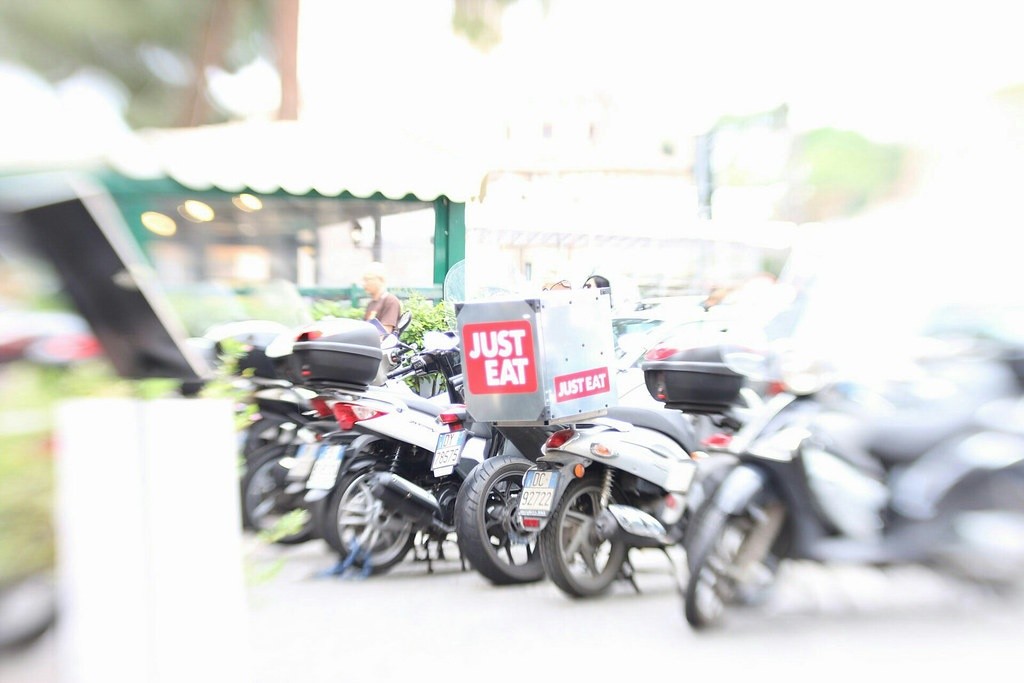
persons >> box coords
[581,274,612,308]
[354,260,402,335]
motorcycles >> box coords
[229,283,1023,636]
